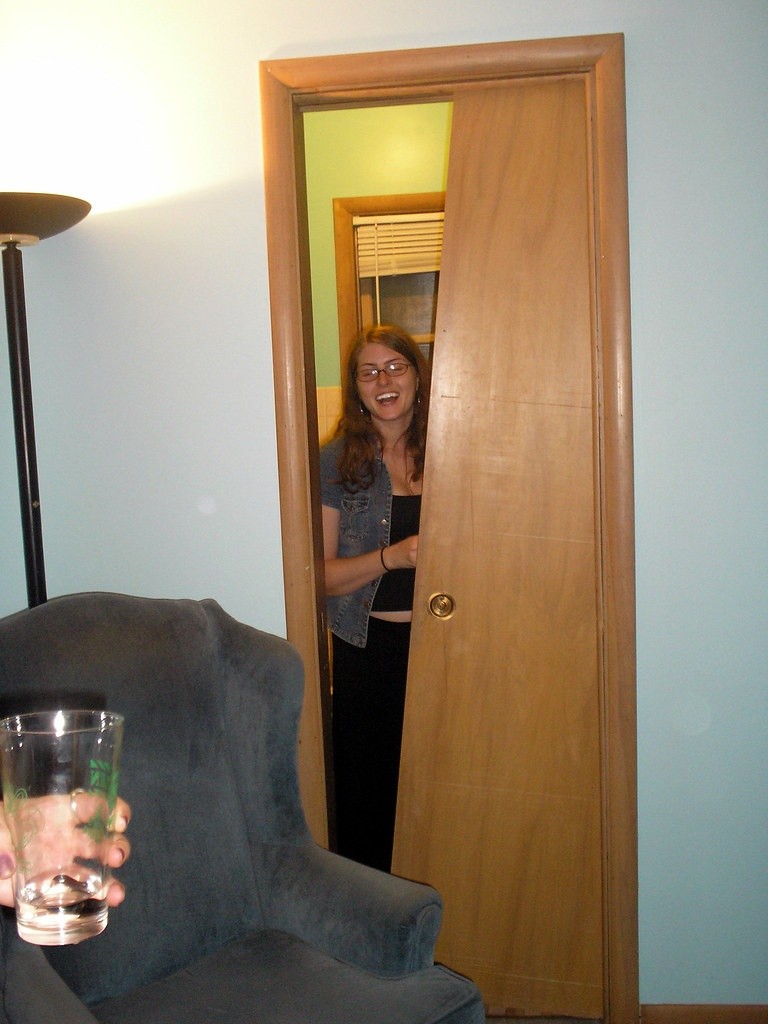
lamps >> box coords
[0,192,90,611]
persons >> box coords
[0,787,133,917]
[316,324,429,875]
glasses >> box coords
[356,362,412,382]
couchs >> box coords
[0,593,485,1024]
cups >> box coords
[0,710,125,945]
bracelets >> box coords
[379,545,392,572]
